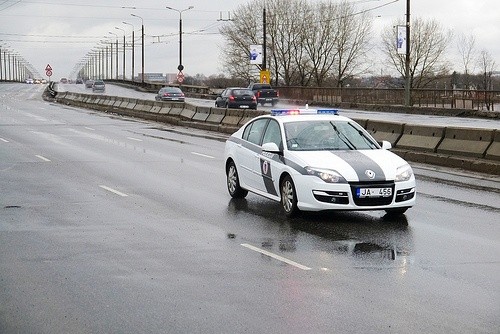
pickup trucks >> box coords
[248,83,280,107]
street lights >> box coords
[123,21,135,81]
[116,26,126,80]
[109,32,118,79]
[74,35,112,80]
[166,5,194,87]
[0,39,40,82]
[131,13,144,82]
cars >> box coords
[85,79,94,88]
[26,78,48,84]
[60,77,83,84]
[92,81,107,93]
[214,86,257,110]
[154,87,185,102]
[223,106,415,216]
